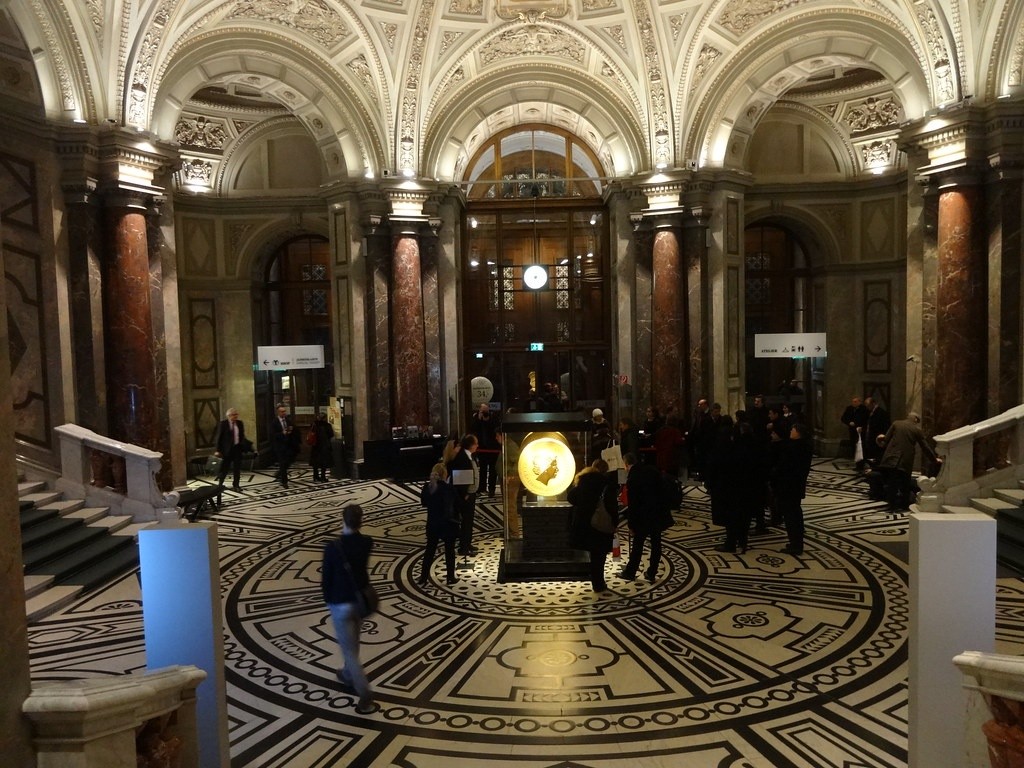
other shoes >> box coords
[356,692,374,709]
[644,571,655,580]
[283,483,289,489]
[713,517,804,553]
[615,572,634,580]
[233,483,242,491]
[459,550,478,556]
[448,578,460,584]
[469,546,478,551]
[274,474,280,479]
[335,670,355,686]
[419,578,427,583]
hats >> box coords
[909,413,921,424]
[592,409,603,418]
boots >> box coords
[313,467,320,481]
[320,469,328,482]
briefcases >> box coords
[206,454,223,474]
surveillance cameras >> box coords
[906,355,914,362]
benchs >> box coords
[174,484,225,520]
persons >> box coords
[419,434,479,584]
[468,405,501,496]
[528,382,559,401]
[215,407,245,492]
[310,413,334,482]
[565,394,812,594]
[274,407,296,486]
[322,504,374,707]
[841,397,938,510]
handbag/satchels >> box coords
[855,432,863,463]
[355,586,380,617]
[591,486,617,535]
[306,422,316,445]
[601,439,625,472]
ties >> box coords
[232,422,235,440]
[282,418,287,432]
